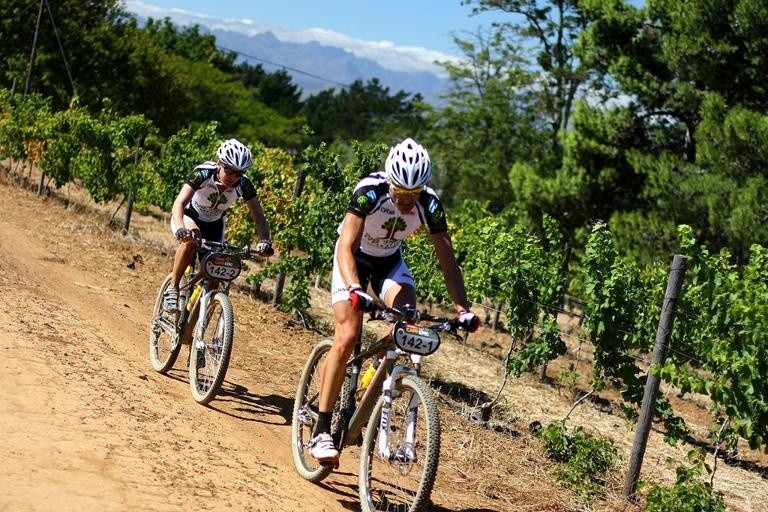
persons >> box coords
[307,137,480,463]
[162,139,274,368]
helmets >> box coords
[215,138,252,170]
[384,138,432,190]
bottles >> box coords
[186,282,202,312]
[359,359,381,401]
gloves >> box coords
[347,284,374,312]
[256,239,274,257]
[175,228,192,243]
[455,310,480,333]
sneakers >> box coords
[197,351,205,367]
[311,433,340,458]
[162,285,179,313]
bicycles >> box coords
[290,285,468,512]
[149,231,272,404]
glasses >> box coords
[221,163,243,177]
[389,179,423,199]
